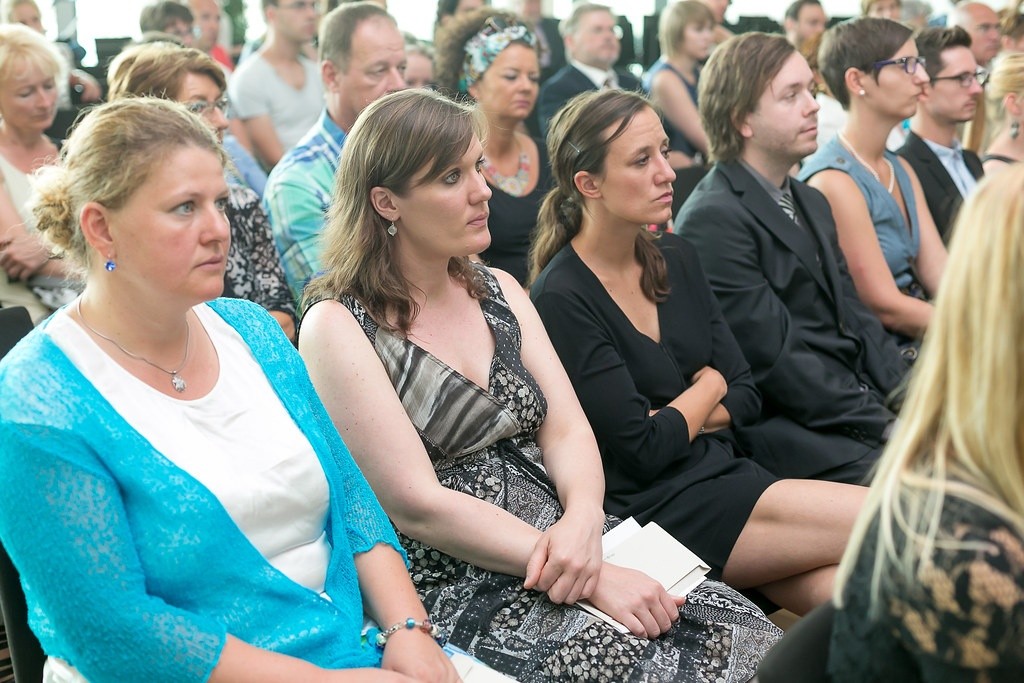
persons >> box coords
[0,0,1024,683]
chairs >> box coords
[0,304,48,683]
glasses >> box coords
[861,57,929,76]
[183,97,229,117]
[936,64,987,88]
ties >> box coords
[777,190,802,226]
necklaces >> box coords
[481,135,530,196]
[836,129,895,194]
[77,296,191,393]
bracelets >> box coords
[698,427,704,434]
[377,618,447,649]
